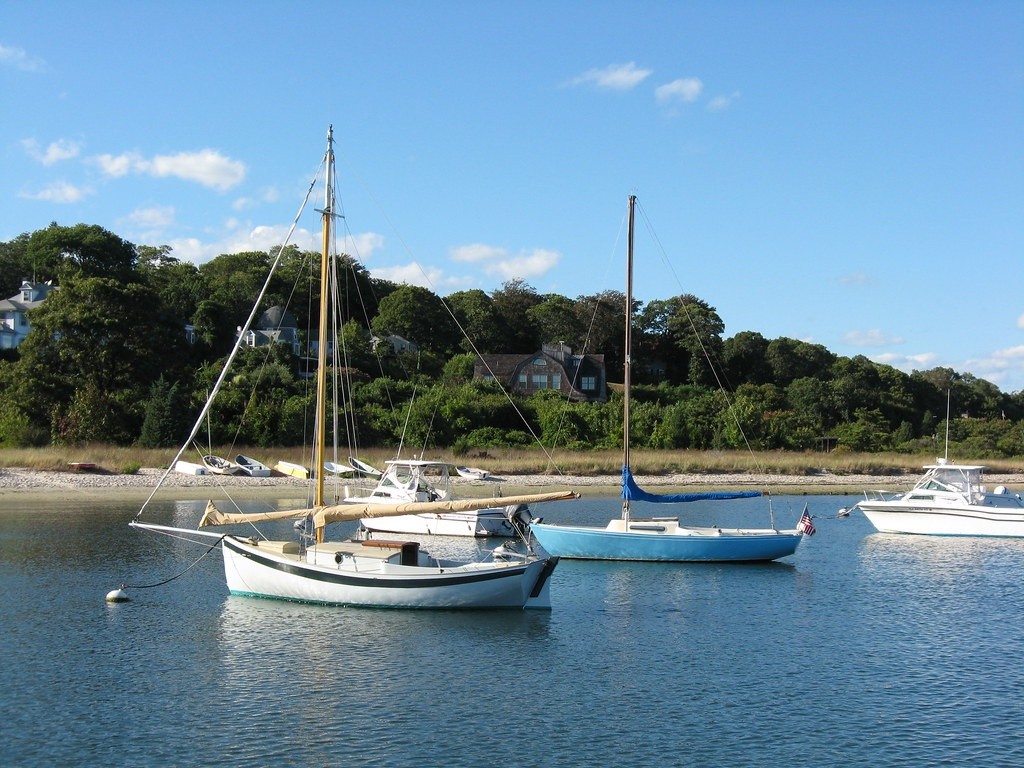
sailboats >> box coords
[526,194,829,562]
[123,123,583,611]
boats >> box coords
[454,466,493,481]
[856,389,1024,540]
[347,456,384,481]
[235,454,271,477]
[323,462,355,479]
[203,455,241,474]
[273,459,310,481]
[175,460,207,475]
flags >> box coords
[798,507,816,536]
